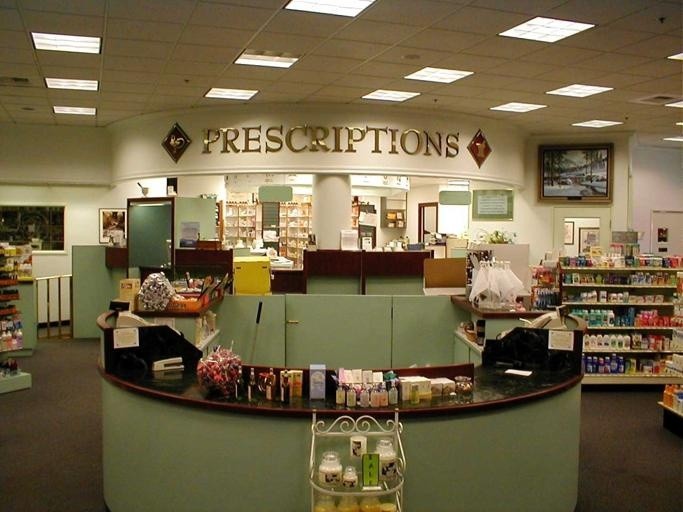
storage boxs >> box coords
[167,276,229,314]
[233,256,272,292]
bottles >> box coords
[572,290,631,373]
[234,365,244,401]
[0,328,25,353]
[342,465,358,490]
[248,367,257,401]
[265,367,276,401]
[335,379,398,408]
[280,370,291,403]
[317,450,344,489]
[663,383,683,414]
[375,438,399,482]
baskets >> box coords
[162,297,205,313]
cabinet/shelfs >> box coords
[558,256,683,387]
[215,200,404,267]
[530,250,558,310]
[424,236,530,296]
[659,273,680,436]
[1,243,34,396]
[308,408,406,510]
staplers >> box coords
[152,357,184,371]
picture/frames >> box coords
[536,141,613,204]
[99,207,127,244]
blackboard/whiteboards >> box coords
[127,204,175,271]
